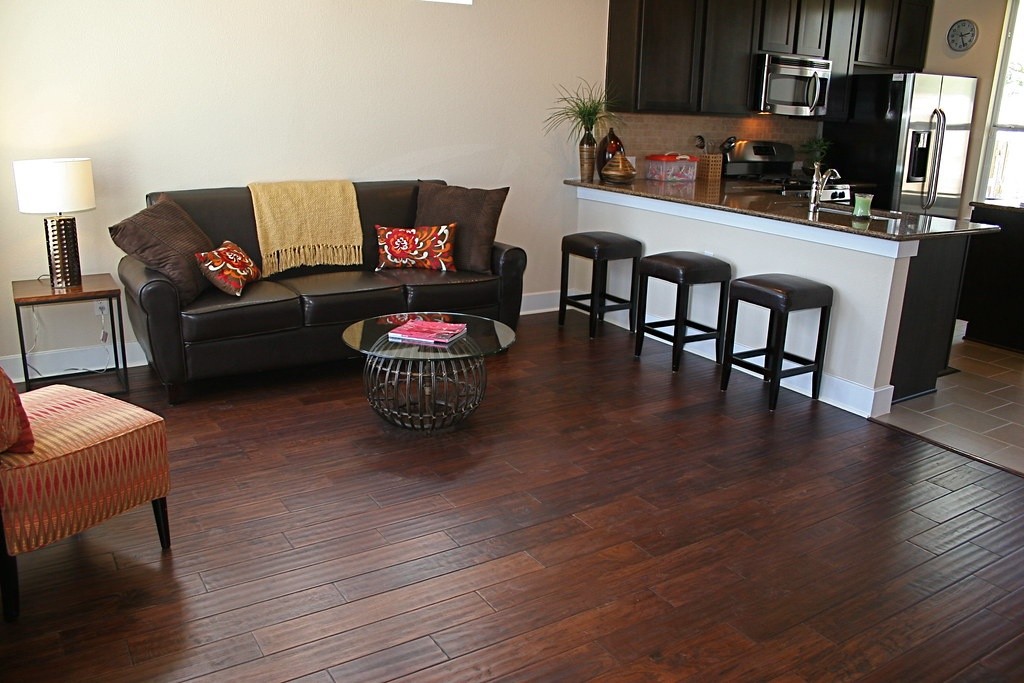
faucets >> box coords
[808,161,841,212]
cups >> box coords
[853,193,874,217]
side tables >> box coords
[14,273,130,399]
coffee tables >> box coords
[342,312,516,432]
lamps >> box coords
[14,157,96,287]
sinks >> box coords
[774,201,913,220]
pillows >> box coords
[197,240,262,295]
[109,192,212,302]
[0,368,36,456]
[414,181,510,276]
[372,223,457,274]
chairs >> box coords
[0,385,172,623]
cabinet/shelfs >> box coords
[858,0,935,72]
[760,0,833,57]
[635,0,756,118]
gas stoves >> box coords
[725,139,851,203]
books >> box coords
[388,319,468,343]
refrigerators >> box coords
[826,72,978,221]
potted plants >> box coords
[543,78,626,182]
[800,136,831,176]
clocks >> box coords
[947,19,978,50]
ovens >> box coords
[752,52,833,117]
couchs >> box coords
[119,180,527,406]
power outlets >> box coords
[94,298,109,316]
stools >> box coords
[559,230,642,340]
[634,251,731,370]
[720,274,834,411]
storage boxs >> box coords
[645,152,699,181]
